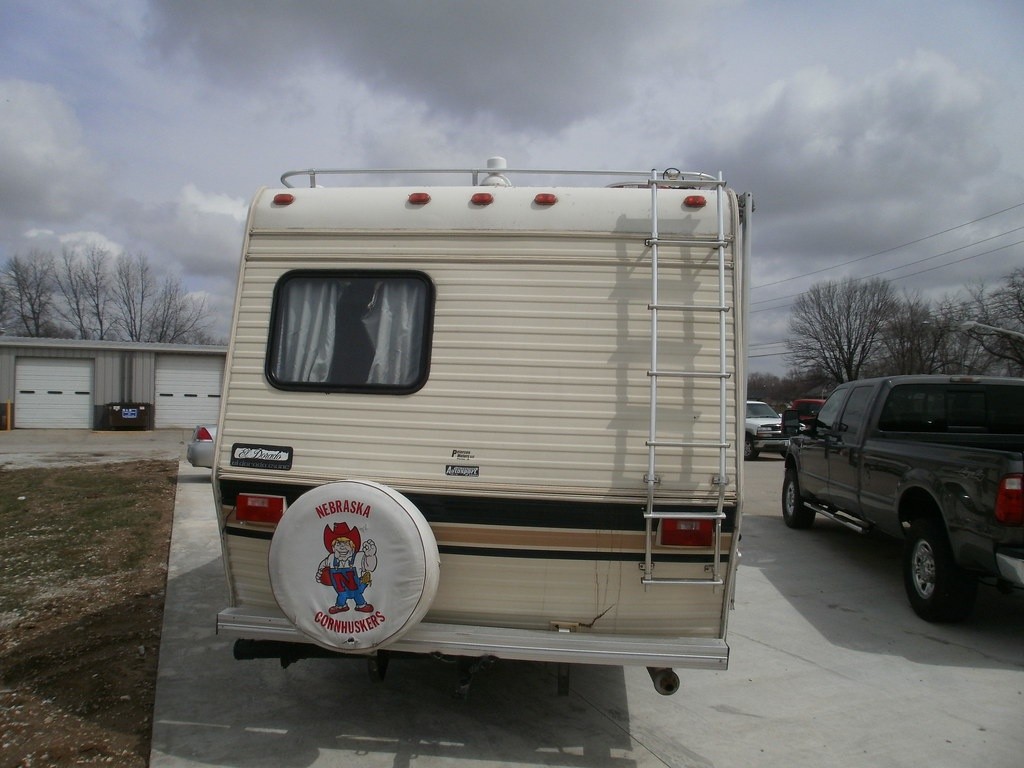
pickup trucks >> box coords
[781,373,1024,627]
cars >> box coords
[745,399,792,461]
[187,423,218,468]
[783,398,829,436]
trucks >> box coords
[212,158,757,701]
